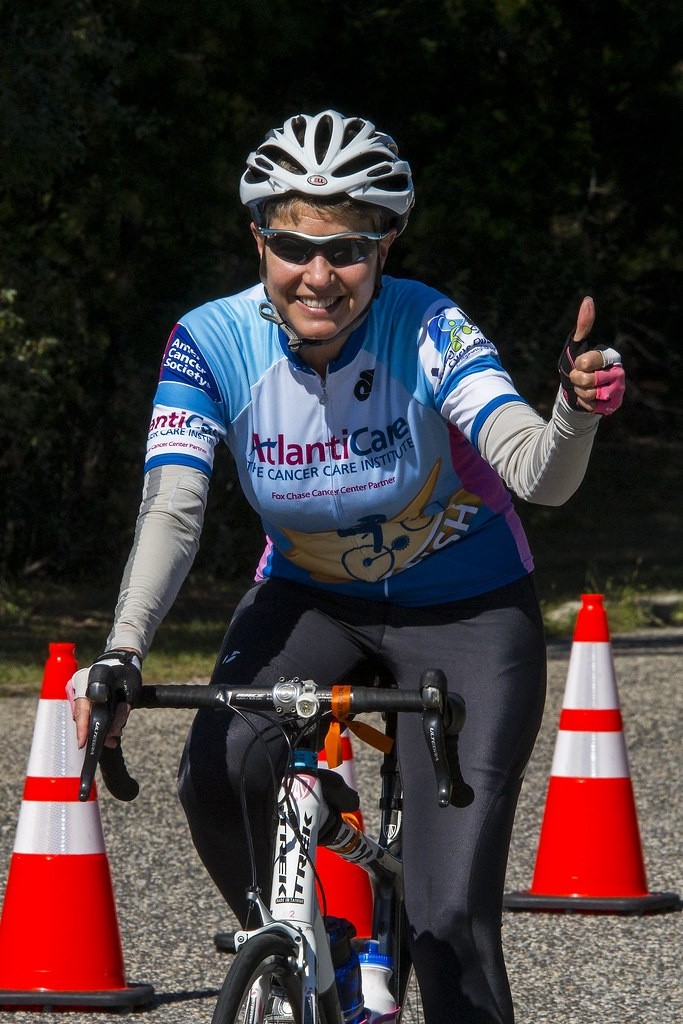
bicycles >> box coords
[78,663,478,1024]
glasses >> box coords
[254,222,390,268]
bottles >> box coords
[358,940,396,1024]
[323,915,364,1024]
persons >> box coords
[64,110,624,1024]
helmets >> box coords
[239,110,416,239]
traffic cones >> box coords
[501,596,681,915]
[0,642,157,1011]
